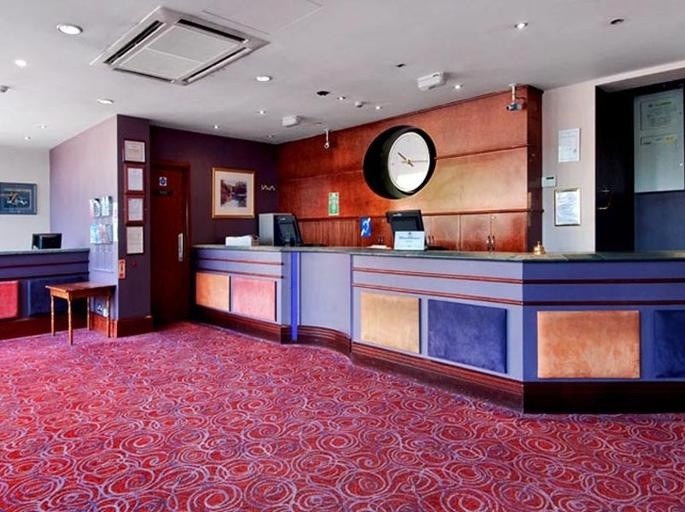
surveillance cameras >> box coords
[505,103,523,112]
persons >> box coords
[222,189,239,208]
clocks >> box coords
[387,130,433,195]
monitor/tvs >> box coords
[386,209,427,248]
[32,233,63,249]
[274,215,305,246]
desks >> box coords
[45,281,116,345]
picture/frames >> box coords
[557,128,581,163]
[553,188,580,227]
[0,183,38,215]
[210,168,255,220]
[120,139,147,256]
